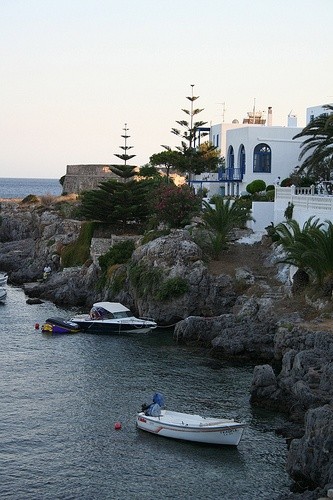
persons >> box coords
[141,393,164,416]
[274,176,280,185]
[43,263,52,282]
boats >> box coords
[70,302,157,335]
[0,272,8,286]
[0,287,7,302]
[136,409,248,447]
